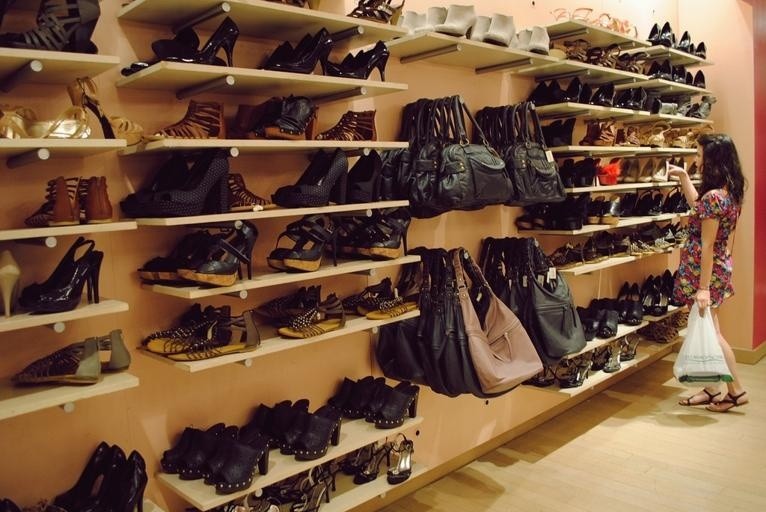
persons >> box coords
[666,133,749,413]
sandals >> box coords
[185,494,283,512]
[0,0,102,55]
[314,109,378,141]
[0,104,92,139]
[277,291,347,338]
[648,189,664,216]
[167,309,262,361]
[346,0,405,26]
[661,187,682,213]
[671,310,690,329]
[10,336,101,385]
[705,391,750,413]
[648,121,671,147]
[655,326,680,343]
[153,99,227,140]
[624,51,650,73]
[23,175,83,228]
[672,131,696,148]
[587,195,606,225]
[600,195,621,225]
[355,215,373,257]
[229,173,277,212]
[253,284,322,319]
[678,388,722,406]
[100,329,131,372]
[565,38,592,63]
[78,175,114,225]
[615,52,631,70]
[597,42,622,69]
[584,45,603,65]
[365,295,419,320]
[140,302,231,355]
[342,276,393,314]
[634,190,652,215]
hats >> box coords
[151,38,228,67]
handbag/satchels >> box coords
[519,236,562,367]
[454,249,520,400]
[415,248,443,395]
[454,246,545,395]
[378,98,446,219]
[375,245,430,387]
[399,95,486,212]
[425,248,461,399]
[442,250,472,395]
[438,94,515,211]
[503,100,568,208]
[525,237,587,358]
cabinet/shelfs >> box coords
[2,0,715,512]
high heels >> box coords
[215,432,274,493]
[66,75,143,146]
[620,332,640,361]
[326,60,344,77]
[434,4,478,40]
[0,249,21,319]
[617,160,632,183]
[637,158,657,183]
[240,399,293,434]
[327,375,375,410]
[668,156,689,182]
[202,428,261,483]
[386,433,415,485]
[176,220,259,286]
[374,385,420,429]
[645,22,662,45]
[652,157,670,182]
[279,461,340,502]
[259,397,310,438]
[626,85,648,110]
[615,281,631,323]
[676,64,688,84]
[393,11,427,40]
[164,16,240,68]
[353,441,395,485]
[160,422,226,475]
[570,157,594,185]
[289,465,334,512]
[534,78,561,106]
[270,148,329,208]
[53,441,111,512]
[626,283,643,325]
[177,424,240,480]
[284,147,349,207]
[534,358,564,387]
[653,283,672,316]
[545,195,576,230]
[370,207,412,259]
[617,87,634,108]
[579,82,593,104]
[127,450,146,471]
[27,236,104,313]
[342,439,379,475]
[558,353,594,388]
[562,77,584,103]
[342,39,391,82]
[625,158,640,183]
[18,239,95,310]
[282,213,340,272]
[267,26,335,76]
[526,79,547,101]
[659,22,674,47]
[562,192,590,230]
[80,443,127,512]
[676,31,692,52]
[362,379,411,421]
[350,148,382,203]
[413,6,448,35]
[150,151,231,217]
[136,229,243,285]
[293,408,344,460]
[641,275,663,314]
[263,94,320,140]
[343,376,385,420]
[267,220,300,272]
[695,41,707,60]
[470,15,492,42]
[527,26,550,55]
[603,337,623,373]
[130,27,201,71]
[694,69,706,88]
[276,403,335,453]
[482,12,516,47]
[658,58,673,80]
[596,157,624,185]
[516,29,532,51]
[597,82,617,107]
[116,459,149,512]
[646,60,661,80]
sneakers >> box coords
[692,95,717,119]
[533,204,550,228]
[630,240,665,256]
[578,123,601,146]
[549,241,584,270]
[599,309,620,338]
[516,214,533,229]
[593,121,617,147]
[651,98,679,114]
